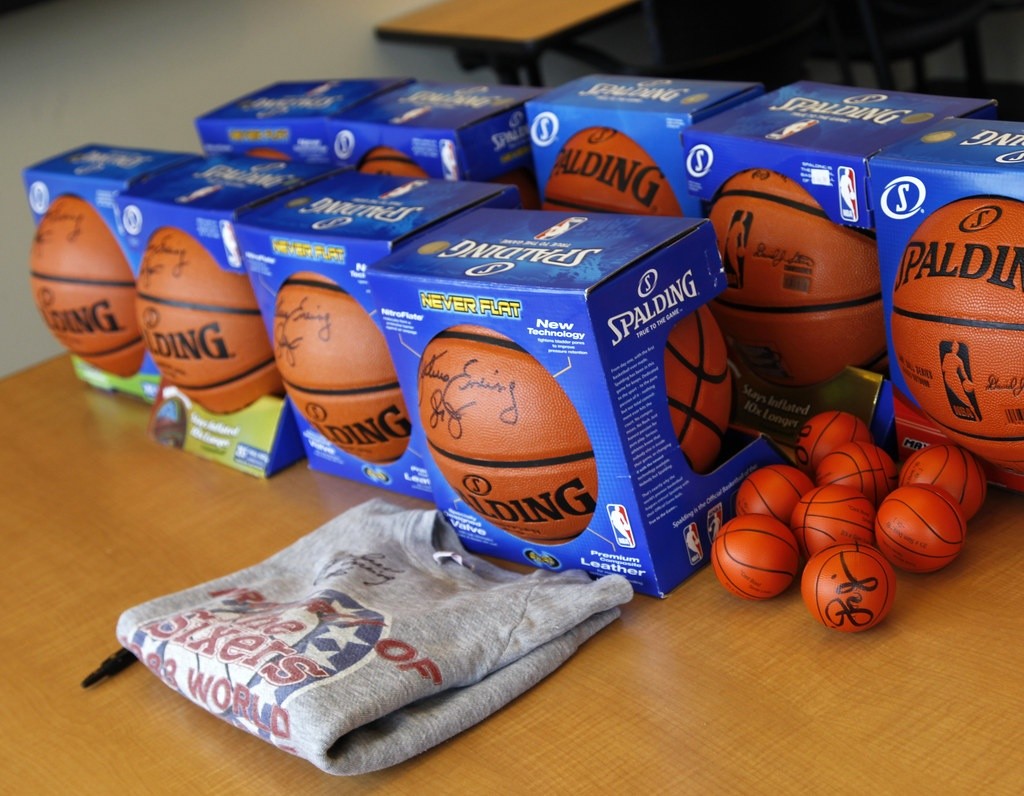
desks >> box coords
[0,350,1024,796]
[375,0,654,84]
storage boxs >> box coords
[230,170,519,502]
[367,210,787,599]
[867,114,1024,494]
[118,155,352,473]
[195,77,417,166]
[678,80,1001,450]
[335,81,541,210]
[24,143,208,402]
[526,73,764,215]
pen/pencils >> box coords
[78,645,138,689]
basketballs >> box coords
[357,146,539,213]
[706,166,891,389]
[890,196,1024,477]
[28,196,149,380]
[710,405,983,635]
[130,229,286,415]
[538,123,689,218]
[375,212,732,548]
[266,267,410,470]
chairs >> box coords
[643,0,820,83]
[810,0,992,95]
